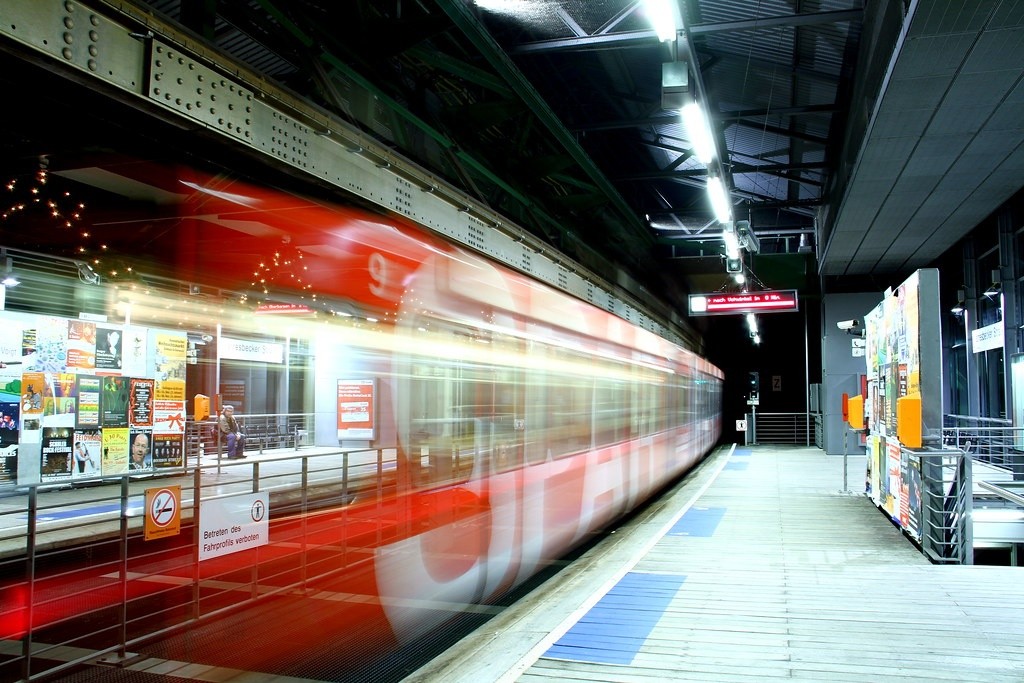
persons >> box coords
[64,400,74,413]
[101,331,121,368]
[219,406,246,459]
[129,433,150,470]
[0,411,17,430]
[75,442,90,473]
[44,400,60,416]
[62,374,76,396]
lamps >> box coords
[660,32,697,109]
[985,286,998,296]
[951,302,964,313]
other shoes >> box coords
[238,455,247,458]
[228,456,238,459]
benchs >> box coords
[210,423,302,460]
